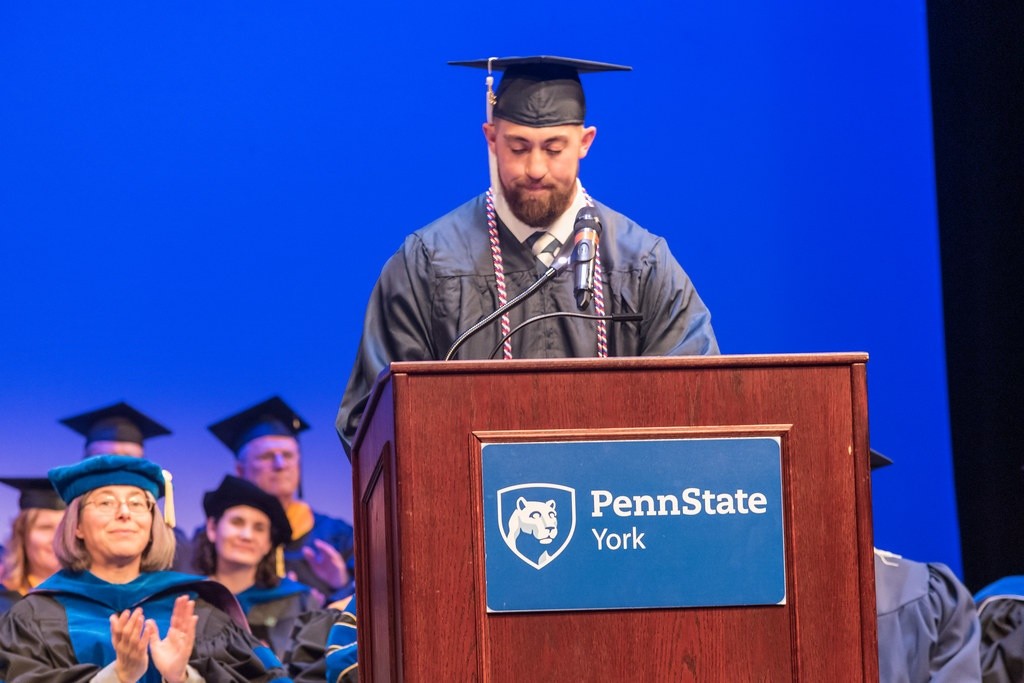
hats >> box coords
[446,54,633,208]
[208,395,310,497]
[59,401,172,447]
[202,474,293,580]
[0,478,69,511]
[47,454,176,526]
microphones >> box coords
[571,206,602,311]
[488,312,645,360]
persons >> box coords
[334,54,720,465]
[0,398,359,683]
[870,452,1024,683]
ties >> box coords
[523,231,562,269]
